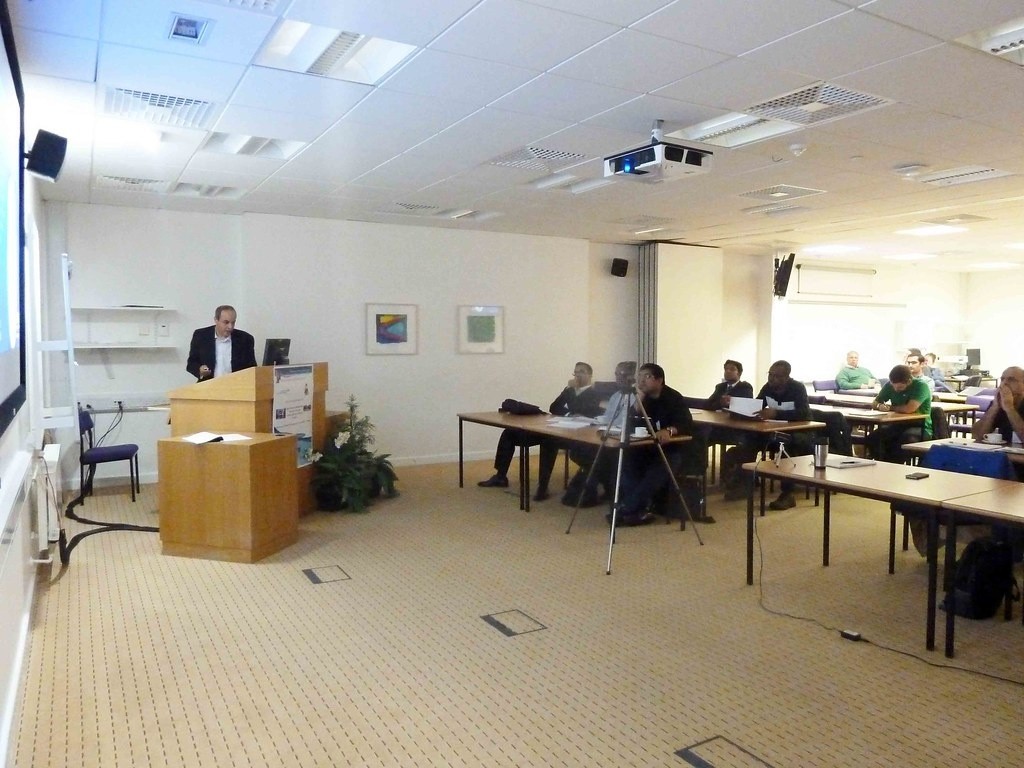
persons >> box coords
[705,359,752,445]
[971,365,1024,445]
[186,305,258,383]
[866,365,933,464]
[905,346,944,402]
[474,362,600,502]
[836,350,881,396]
[563,360,701,528]
[722,360,814,509]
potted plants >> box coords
[317,393,399,512]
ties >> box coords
[728,386,732,389]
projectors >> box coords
[603,141,713,185]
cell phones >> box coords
[905,472,928,479]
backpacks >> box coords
[939,537,1020,619]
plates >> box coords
[631,433,651,438]
[982,440,1007,445]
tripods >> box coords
[565,376,706,575]
[773,441,796,467]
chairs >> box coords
[78,401,140,503]
[588,375,1004,574]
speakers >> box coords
[611,258,629,277]
[26,129,67,182]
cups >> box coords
[983,433,1002,442]
[635,427,648,436]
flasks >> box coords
[814,436,829,469]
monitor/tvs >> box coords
[774,253,795,296]
[262,339,291,366]
[966,349,980,371]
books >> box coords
[723,396,764,420]
[183,432,253,445]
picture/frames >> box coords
[456,305,505,356]
[366,303,417,355]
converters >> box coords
[842,628,861,640]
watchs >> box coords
[876,403,882,409]
[666,427,673,436]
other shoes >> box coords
[477,475,508,487]
[769,493,797,510]
[739,476,759,488]
[606,506,635,526]
[533,488,549,500]
[635,512,655,523]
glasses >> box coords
[615,371,626,376]
[638,374,655,379]
[572,372,583,377]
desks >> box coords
[598,401,827,511]
[458,412,693,531]
[943,375,1000,392]
[806,404,930,469]
[846,390,973,437]
[806,392,979,443]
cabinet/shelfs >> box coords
[894,439,1024,482]
[738,452,1024,668]
[68,306,179,350]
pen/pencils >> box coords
[842,462,858,463]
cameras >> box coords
[616,361,636,376]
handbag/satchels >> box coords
[657,478,703,522]
[561,468,597,507]
[499,399,541,415]
